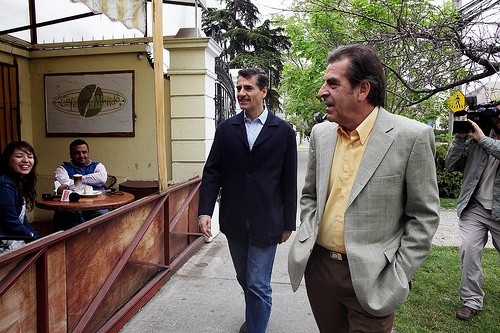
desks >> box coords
[35,190,135,227]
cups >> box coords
[68,193,80,201]
[84,186,93,194]
[73,174,82,186]
[41,193,51,200]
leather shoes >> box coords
[456,305,479,319]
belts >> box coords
[315,244,348,263]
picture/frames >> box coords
[43,69,136,138]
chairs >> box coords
[105,176,117,189]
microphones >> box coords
[453,110,467,117]
[42,189,80,202]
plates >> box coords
[76,191,102,197]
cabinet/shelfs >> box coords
[119,181,159,200]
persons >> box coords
[52,139,109,230]
[197,67,298,333]
[0,140,39,253]
[287,45,440,333]
[445,100,500,321]
